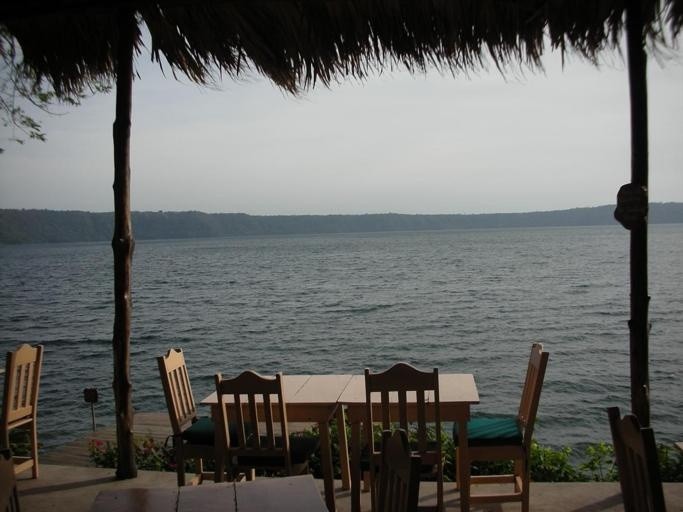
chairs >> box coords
[212,366,312,481]
[361,362,449,510]
[452,342,549,511]
[607,406,666,512]
[0,344,44,512]
[156,346,256,488]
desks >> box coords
[198,374,350,511]
[90,473,328,512]
[337,374,482,511]
